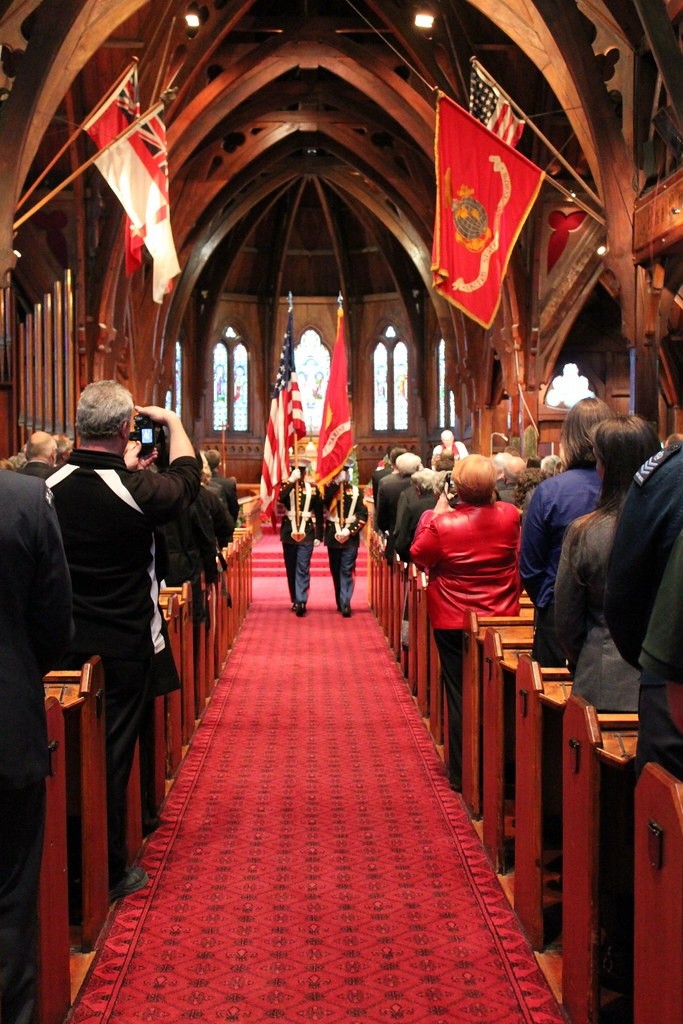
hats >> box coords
[289,458,311,472]
[342,457,354,470]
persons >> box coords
[151,445,240,589]
[601,434,683,780]
[552,416,661,714]
[492,452,562,511]
[324,457,368,617]
[370,443,455,566]
[0,430,69,478]
[277,457,324,616]
[0,471,72,1024]
[431,430,469,471]
[412,454,524,792]
[44,379,202,900]
[519,397,617,669]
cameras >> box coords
[129,414,166,456]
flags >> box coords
[92,103,182,305]
[315,309,355,499]
[431,93,545,329]
[260,311,306,522]
[84,63,145,278]
[469,65,526,149]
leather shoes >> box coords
[292,602,307,616]
[338,606,350,617]
[109,867,151,902]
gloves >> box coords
[289,470,300,483]
[340,529,350,539]
[333,471,345,485]
[313,539,320,547]
